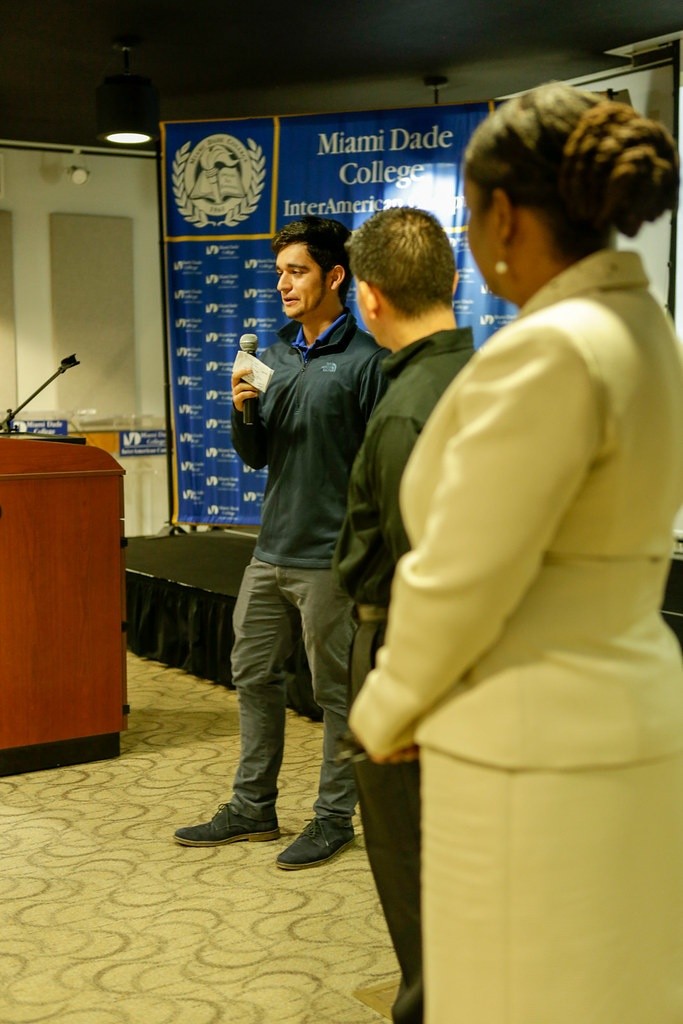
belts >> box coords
[356,602,389,621]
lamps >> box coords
[95,72,158,146]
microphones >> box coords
[239,334,258,426]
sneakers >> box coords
[276,819,354,871]
[173,809,279,847]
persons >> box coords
[175,216,397,872]
[316,204,482,1023]
[350,79,682,1024]
